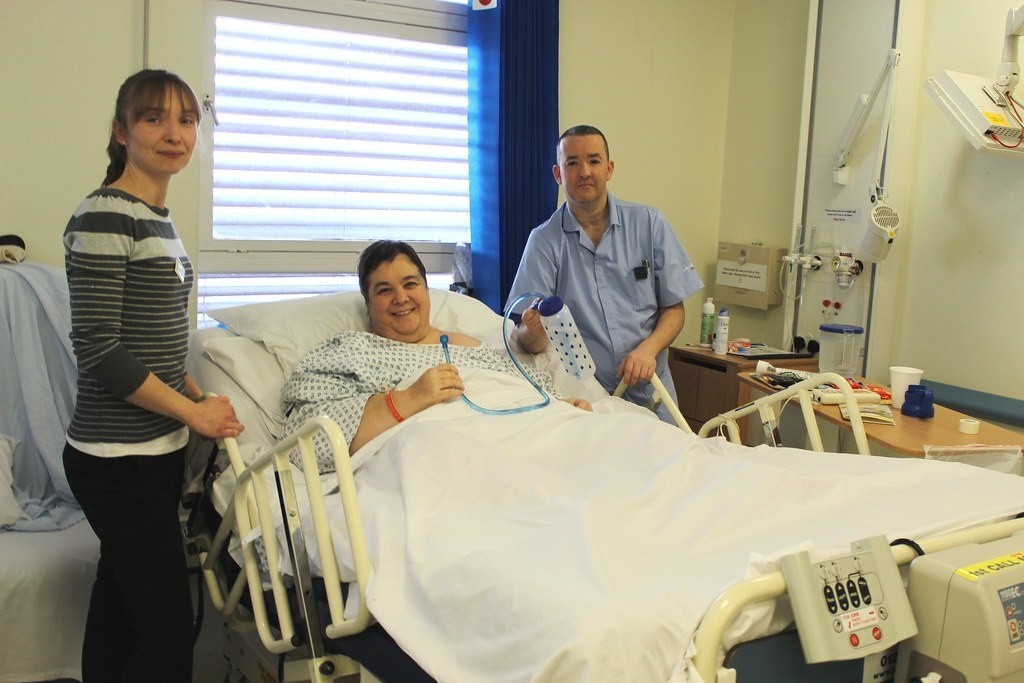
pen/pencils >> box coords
[645,259,651,270]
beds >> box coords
[182,329,1023,682]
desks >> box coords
[736,370,1023,475]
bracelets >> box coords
[385,390,404,423]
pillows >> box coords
[202,330,292,441]
[200,285,514,385]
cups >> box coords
[889,367,923,409]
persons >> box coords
[503,125,706,429]
[279,240,593,475]
[60,68,245,683]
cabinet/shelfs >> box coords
[664,343,820,449]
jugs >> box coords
[819,324,864,379]
